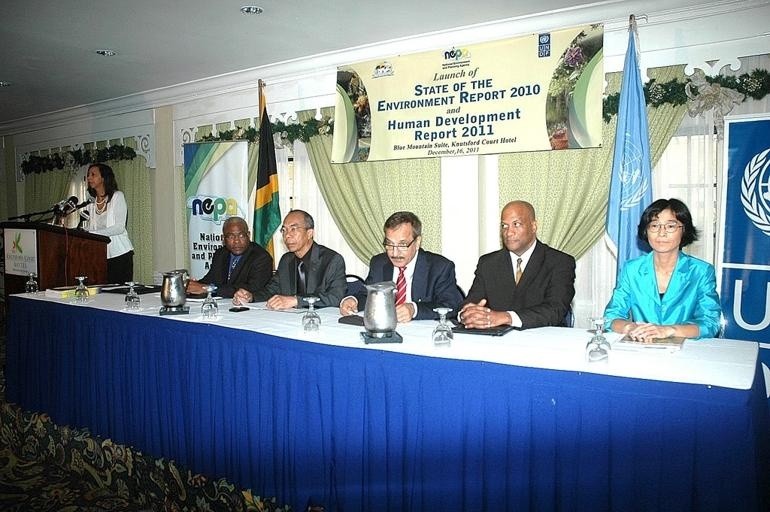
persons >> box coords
[80,163,134,285]
[186,218,273,298]
[340,212,463,322]
[233,210,346,311]
[602,199,722,343]
[454,200,573,330]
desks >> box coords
[6,284,759,512]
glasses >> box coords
[280,227,306,234]
[647,223,682,233]
[222,233,248,241]
[383,237,415,251]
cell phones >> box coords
[229,307,249,312]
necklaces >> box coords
[95,196,106,215]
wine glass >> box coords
[24,271,39,296]
[585,317,613,368]
[200,285,219,320]
[300,296,322,335]
[123,281,140,311]
[431,307,454,351]
[74,275,91,304]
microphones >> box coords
[50,196,92,228]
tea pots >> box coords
[363,279,398,339]
[158,268,191,310]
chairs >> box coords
[345,274,365,296]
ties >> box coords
[230,257,238,272]
[298,259,307,296]
[516,258,522,285]
[395,267,407,306]
